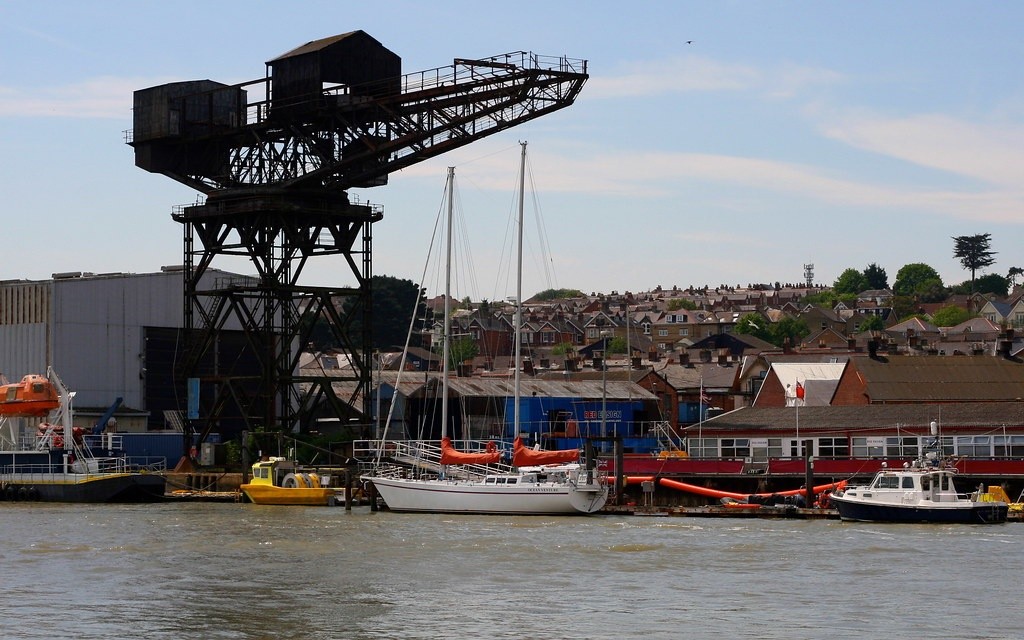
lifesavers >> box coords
[819,493,829,508]
[68,455,73,464]
[190,448,197,460]
[486,441,497,453]
[53,436,63,447]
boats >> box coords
[0,371,61,419]
[827,404,1010,526]
[970,482,1024,523]
[242,435,362,507]
[0,365,168,503]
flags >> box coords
[699,385,712,404]
[797,382,804,402]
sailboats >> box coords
[361,140,610,517]
